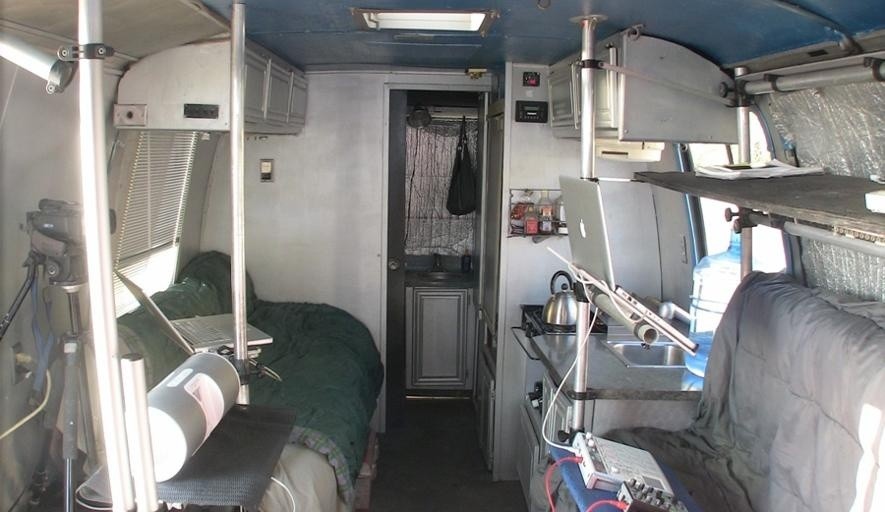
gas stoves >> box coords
[519,304,608,338]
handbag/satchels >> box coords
[447,117,476,215]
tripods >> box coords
[25,332,98,512]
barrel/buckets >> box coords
[682,228,740,381]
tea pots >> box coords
[542,270,579,329]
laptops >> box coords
[558,174,699,353]
[114,268,274,356]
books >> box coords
[695,157,826,180]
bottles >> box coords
[525,389,543,409]
[509,189,570,236]
[461,248,472,274]
